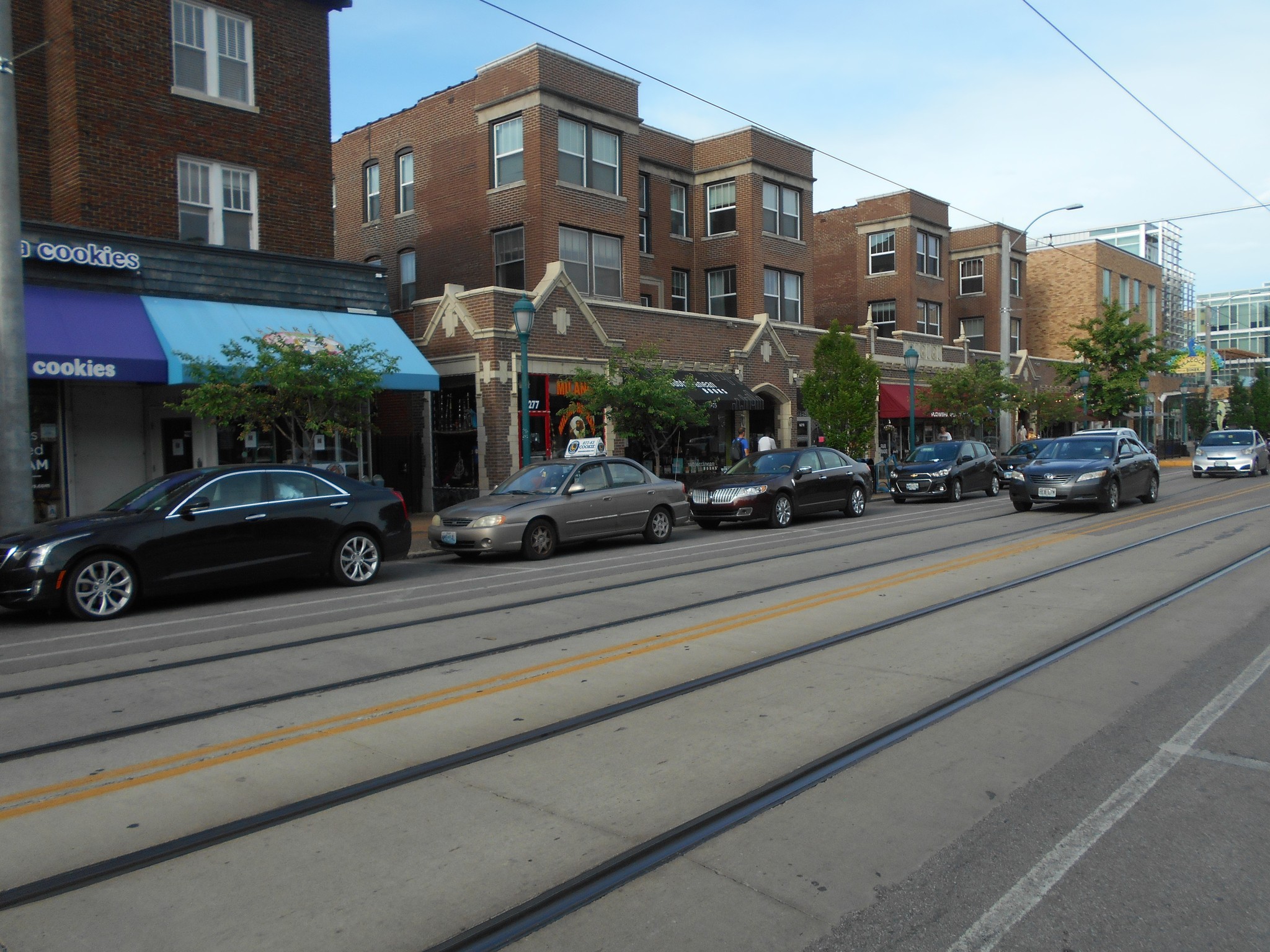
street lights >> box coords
[1000,204,1086,457]
[509,294,544,469]
[903,346,919,456]
[1179,382,1192,445]
[1203,292,1263,435]
[1079,368,1092,429]
[1138,375,1150,443]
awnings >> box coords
[621,366,764,414]
[21,279,441,394]
[878,383,972,417]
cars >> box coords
[1192,430,1270,478]
[998,438,1054,489]
[1010,436,1162,514]
[1,462,413,623]
[426,436,693,561]
[1071,429,1144,460]
[891,440,1000,504]
[687,446,874,529]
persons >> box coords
[938,426,952,441]
[732,427,749,459]
[1017,425,1036,443]
[569,419,586,439]
[758,427,777,452]
